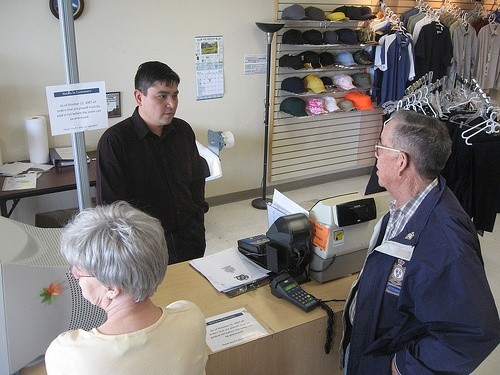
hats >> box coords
[281,4,377,21]
[279,49,374,69]
[280,72,376,92]
[282,28,377,45]
[279,92,374,116]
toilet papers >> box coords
[25,115,49,165]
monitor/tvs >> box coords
[0,216,106,375]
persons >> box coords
[336,110,500,375]
[44,202,208,375]
[94,61,209,267]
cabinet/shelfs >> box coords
[277,18,380,118]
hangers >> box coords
[381,69,500,145]
[379,0,500,44]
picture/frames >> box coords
[49,0,83,21]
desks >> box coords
[0,151,96,218]
[19,261,360,375]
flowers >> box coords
[40,282,64,306]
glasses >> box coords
[75,269,94,279]
[374,137,400,155]
